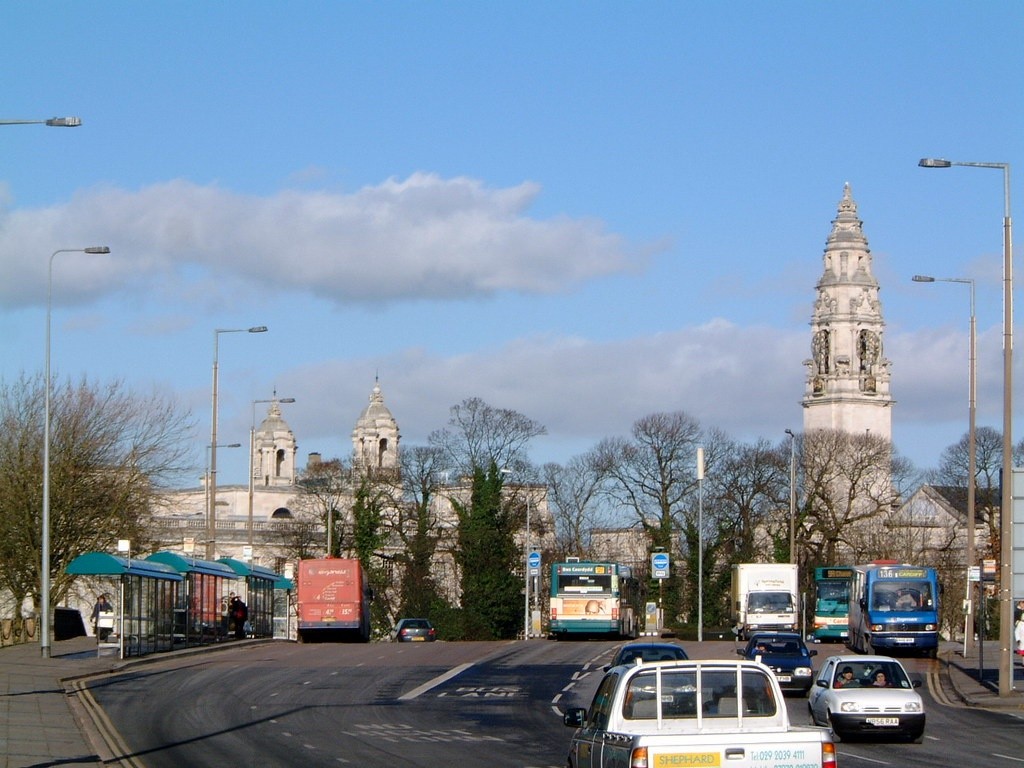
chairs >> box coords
[628,691,658,719]
[717,694,750,718]
[783,642,797,653]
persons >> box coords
[832,666,859,690]
[1014,599,1024,627]
[1013,612,1024,670]
[227,591,236,611]
[756,642,768,653]
[88,595,115,646]
[868,670,896,689]
[229,596,250,640]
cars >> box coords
[605,630,926,742]
[390,618,436,642]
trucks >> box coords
[729,563,804,639]
[294,555,374,642]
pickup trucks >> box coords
[564,655,838,768]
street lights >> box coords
[38,243,296,658]
[782,154,1016,694]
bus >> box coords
[548,557,642,639]
